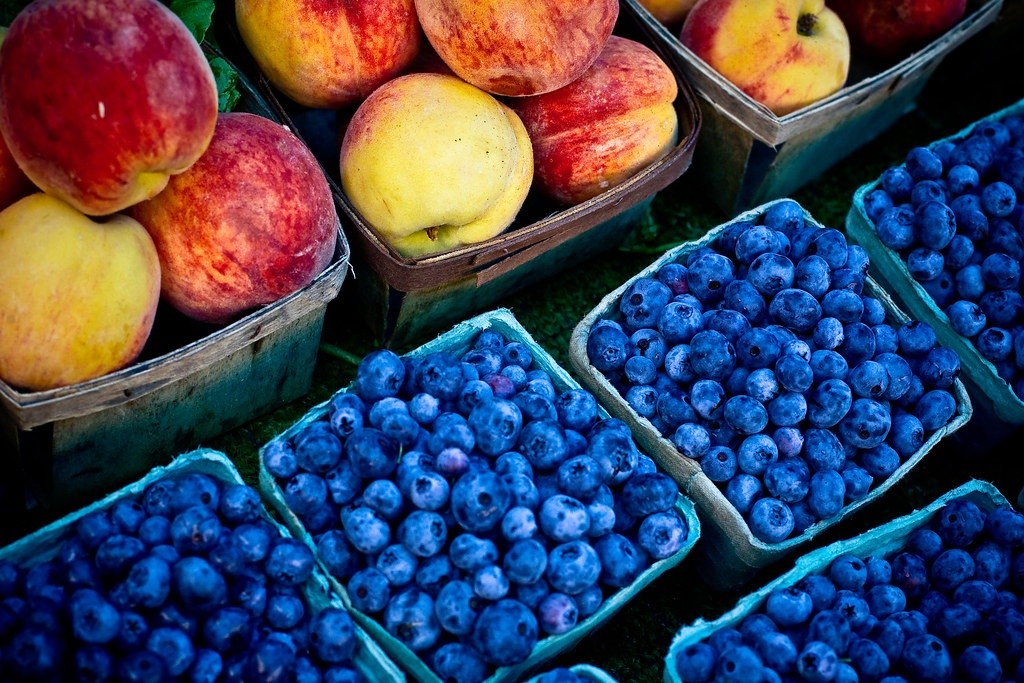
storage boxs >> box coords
[657,477,1024,683]
[524,662,623,683]
[621,0,1006,212]
[222,12,706,356]
[0,38,351,496]
[845,95,1024,425]
[565,190,976,574]
[0,445,409,683]
[256,306,701,683]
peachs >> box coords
[0,0,338,390]
[233,0,678,258]
[638,0,969,117]
